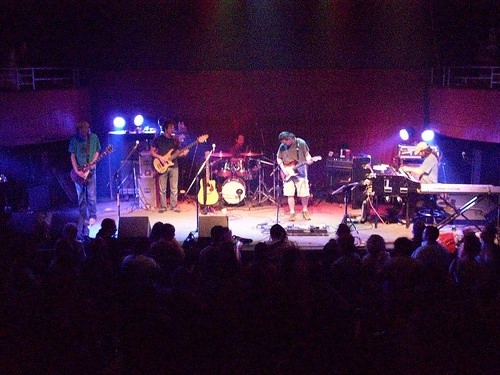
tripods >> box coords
[249,156,277,206]
[332,182,359,234]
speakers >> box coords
[138,152,162,210]
[117,216,151,247]
[199,215,228,244]
[8,210,84,238]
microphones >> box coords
[239,190,241,198]
[212,144,216,152]
[134,140,139,149]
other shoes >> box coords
[302,211,311,220]
[170,206,179,211]
[88,218,96,224]
[289,212,296,221]
[159,208,166,212]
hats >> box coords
[414,141,428,155]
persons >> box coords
[229,134,257,185]
[67,121,101,227]
[277,131,315,221]
[0,209,499,375]
[398,141,440,219]
[150,120,190,213]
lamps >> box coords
[420,126,440,143]
[398,126,416,141]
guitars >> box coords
[152,135,208,174]
[197,151,219,206]
[281,156,322,182]
[70,143,113,185]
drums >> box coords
[211,158,233,178]
[236,157,261,177]
[221,176,246,204]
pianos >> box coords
[416,183,500,236]
[359,162,421,229]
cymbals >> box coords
[210,151,234,157]
[238,152,266,157]
